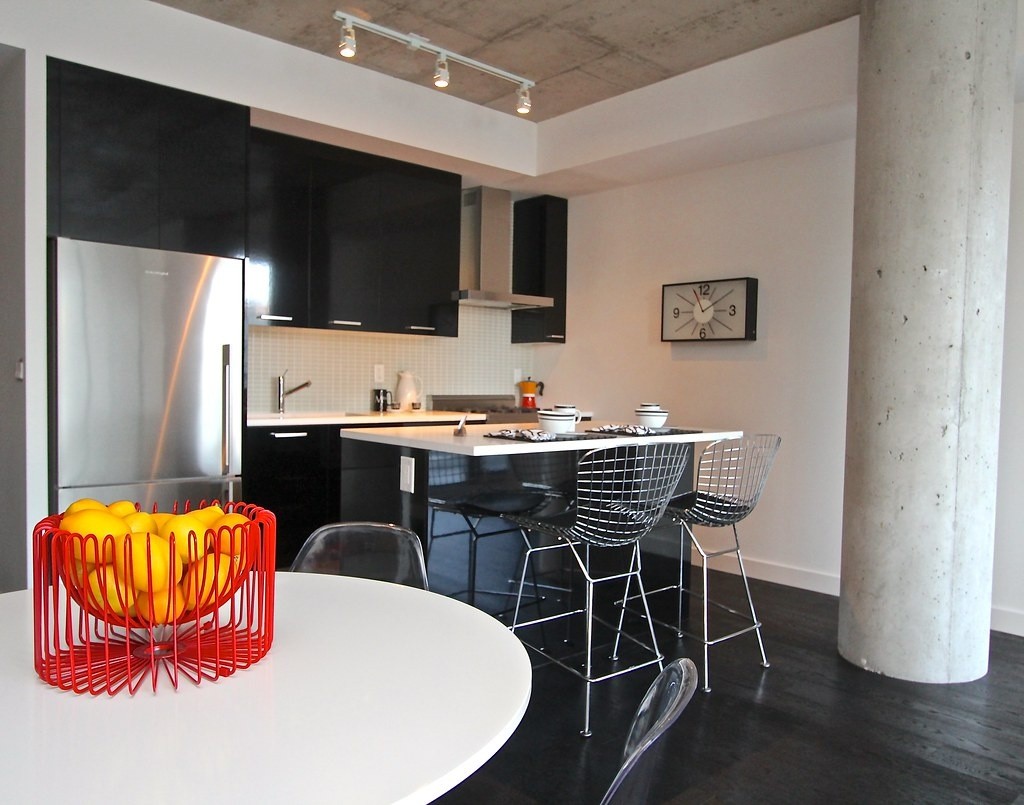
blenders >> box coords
[515,376,544,409]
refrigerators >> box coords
[47,236,245,589]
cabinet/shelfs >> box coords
[48,58,462,345]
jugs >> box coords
[373,389,393,411]
[395,372,423,412]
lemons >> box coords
[57,499,255,623]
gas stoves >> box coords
[427,394,538,424]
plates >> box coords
[649,427,672,432]
[409,410,425,412]
[555,433,589,438]
[386,409,403,413]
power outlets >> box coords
[374,364,385,382]
[514,368,522,384]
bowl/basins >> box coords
[536,410,575,433]
[635,409,669,428]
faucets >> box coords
[278,369,312,414]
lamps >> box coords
[432,53,451,88]
[337,19,358,57]
[515,83,533,114]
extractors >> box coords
[459,186,555,311]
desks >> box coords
[329,422,745,626]
[0,570,536,805]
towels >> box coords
[484,428,556,441]
[584,423,656,436]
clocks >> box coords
[660,275,759,343]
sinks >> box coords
[247,413,373,419]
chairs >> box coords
[290,520,431,592]
[427,472,553,616]
[598,655,700,805]
[508,452,636,593]
[506,440,693,742]
[606,433,783,695]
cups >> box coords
[392,402,400,409]
[412,402,421,410]
[640,403,661,409]
[554,405,582,433]
